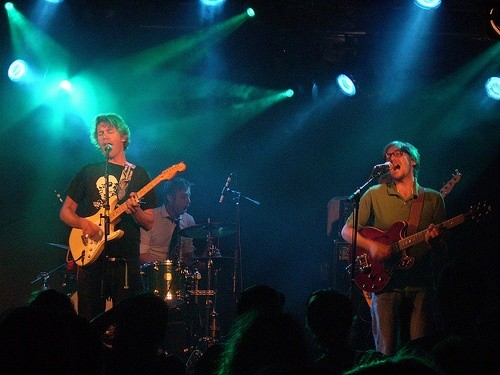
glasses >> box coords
[384,148,409,161]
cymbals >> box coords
[179,220,239,239]
[190,250,234,264]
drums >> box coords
[140,259,187,304]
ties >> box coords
[168,217,181,264]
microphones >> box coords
[104,144,112,151]
[219,173,233,204]
[374,161,392,170]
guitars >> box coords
[343,198,493,294]
[439,168,463,198]
[68,161,187,268]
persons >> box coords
[60,115,159,322]
[0,282,500,375]
[340,141,449,354]
[139,177,205,269]
